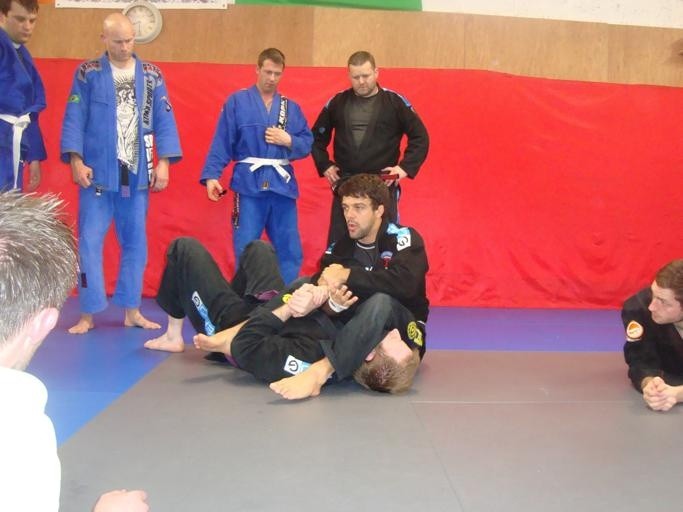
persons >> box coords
[56,11,184,336]
[307,50,432,248]
[0,1,48,196]
[0,184,150,511]
[620,257,683,413]
[190,172,432,400]
[142,237,421,396]
[197,47,315,293]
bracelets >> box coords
[325,297,348,314]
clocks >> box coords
[120,0,163,46]
